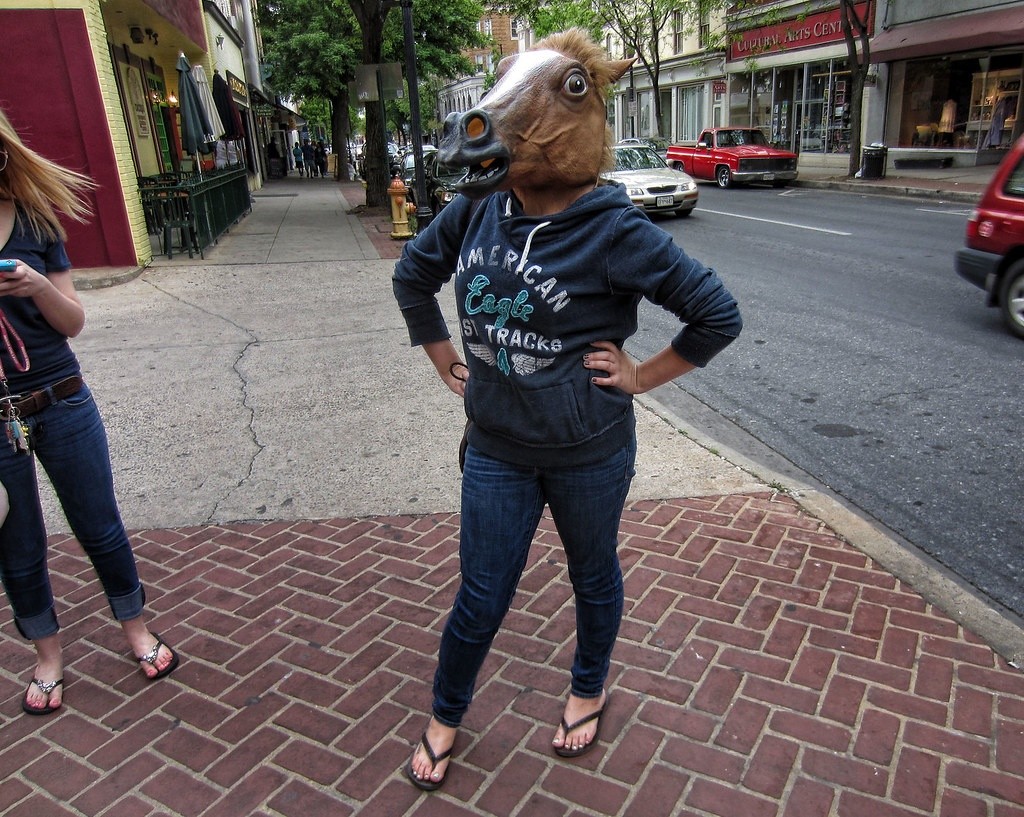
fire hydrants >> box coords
[387,177,417,239]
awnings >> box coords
[857,5,1024,64]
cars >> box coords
[618,138,674,162]
[599,144,699,216]
[349,142,468,218]
[953,133,1024,339]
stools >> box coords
[164,221,199,259]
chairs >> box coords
[136,170,204,235]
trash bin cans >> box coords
[861,145,887,179]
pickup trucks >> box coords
[665,128,798,188]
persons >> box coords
[268,137,281,157]
[938,99,957,144]
[393,29,742,792]
[641,104,649,129]
[0,110,180,716]
[982,97,1008,149]
[213,141,241,170]
[292,139,330,180]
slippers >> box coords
[133,631,180,680]
[406,711,453,789]
[22,664,64,715]
[554,687,609,756]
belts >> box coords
[0,373,83,420]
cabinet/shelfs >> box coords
[821,81,851,153]
[966,69,1024,145]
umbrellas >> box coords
[176,53,225,246]
[212,69,246,169]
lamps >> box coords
[215,32,224,50]
[977,50,990,58]
[645,58,723,83]
[148,87,160,110]
[168,90,178,107]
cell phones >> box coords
[0,260,17,273]
